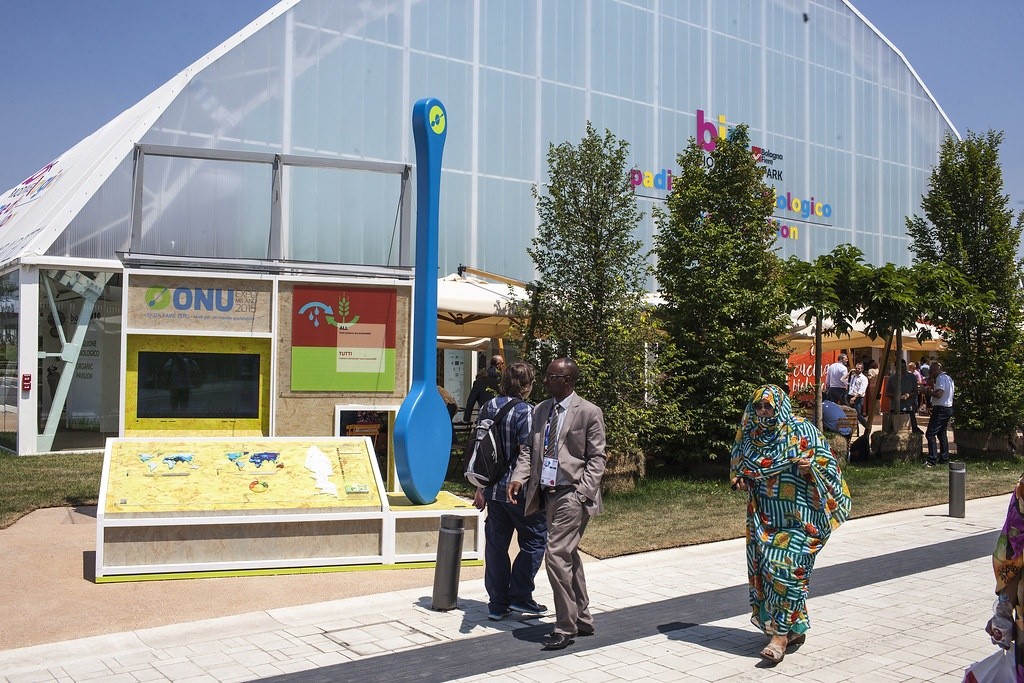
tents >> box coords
[772,310,954,352]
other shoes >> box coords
[924,460,933,468]
[939,459,948,464]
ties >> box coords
[546,403,560,458]
[851,376,856,390]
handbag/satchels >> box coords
[963,643,1017,683]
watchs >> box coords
[999,595,1009,601]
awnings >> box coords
[437,272,593,339]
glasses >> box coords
[542,374,565,381]
[753,402,772,410]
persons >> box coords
[486,354,505,380]
[508,357,607,648]
[730,384,850,662]
[438,386,460,449]
[814,353,956,468]
[463,370,500,422]
[985,471,1024,683]
[472,363,548,619]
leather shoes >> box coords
[577,630,593,636]
[542,633,577,650]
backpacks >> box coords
[464,398,522,489]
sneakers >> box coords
[488,608,511,620]
[509,599,548,614]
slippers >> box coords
[788,632,806,647]
[761,643,785,663]
